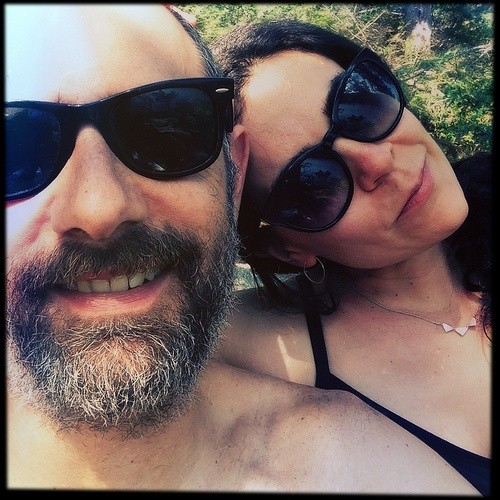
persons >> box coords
[4,4,483,492]
[204,19,495,496]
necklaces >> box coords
[336,266,484,334]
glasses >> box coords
[5,79,235,201]
[247,47,406,235]
